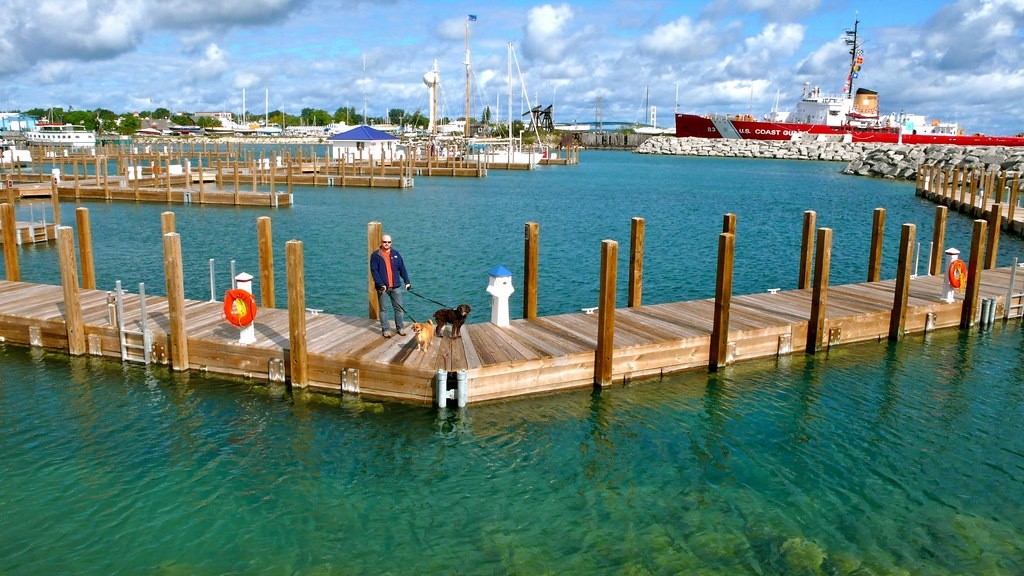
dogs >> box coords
[432,304,472,340]
[410,318,435,354]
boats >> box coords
[26,107,96,147]
[675,12,1024,147]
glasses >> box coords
[382,241,391,243]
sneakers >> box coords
[383,331,391,338]
[397,329,407,336]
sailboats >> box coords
[396,14,545,164]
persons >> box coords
[370,235,411,338]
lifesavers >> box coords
[224,289,258,327]
[949,260,968,288]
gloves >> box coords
[405,283,411,291]
[380,286,386,292]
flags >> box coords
[842,75,852,92]
[467,14,477,21]
[852,56,864,78]
